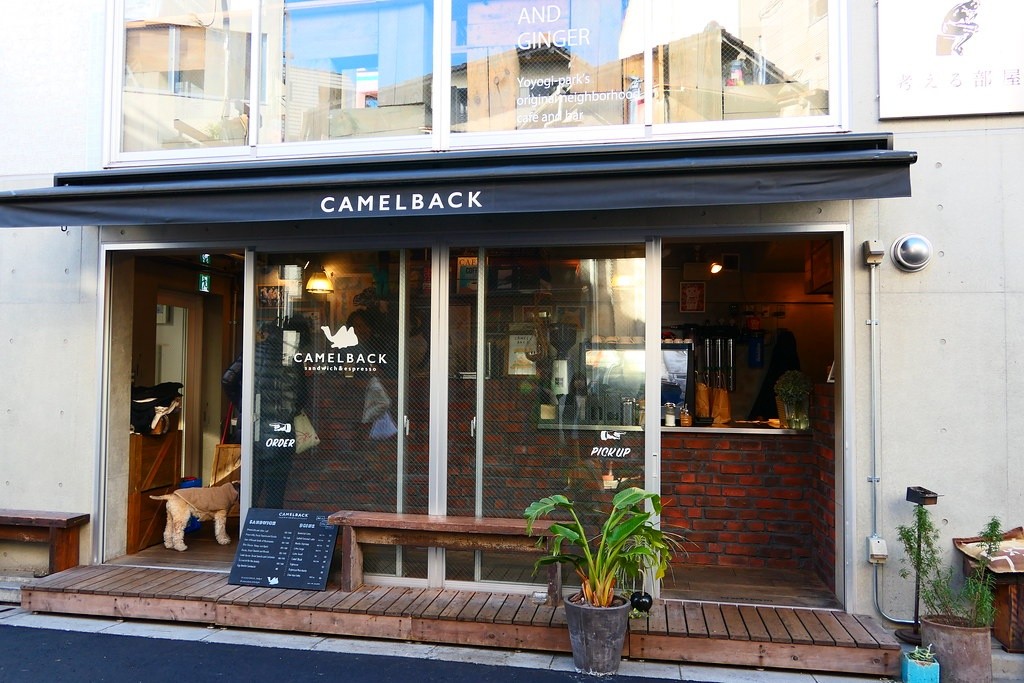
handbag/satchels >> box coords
[294,409,320,454]
[368,410,397,441]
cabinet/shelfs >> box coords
[257,305,325,363]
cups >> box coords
[620,397,635,425]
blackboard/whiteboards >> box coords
[227,506,341,592]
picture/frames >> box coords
[556,305,589,332]
[157,304,170,324]
[521,305,554,322]
[486,304,518,336]
[256,283,288,307]
[679,280,707,314]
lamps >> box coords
[304,264,334,294]
[710,261,723,273]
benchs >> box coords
[0,508,90,575]
[327,512,575,608]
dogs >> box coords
[150,480,242,551]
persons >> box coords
[562,371,603,492]
[219,316,308,509]
[351,365,397,509]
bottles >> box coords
[665,403,676,427]
[639,400,645,425]
[680,403,692,426]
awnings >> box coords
[1,151,918,232]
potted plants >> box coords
[901,643,940,683]
[894,504,1006,683]
[522,487,698,674]
[773,369,815,429]
